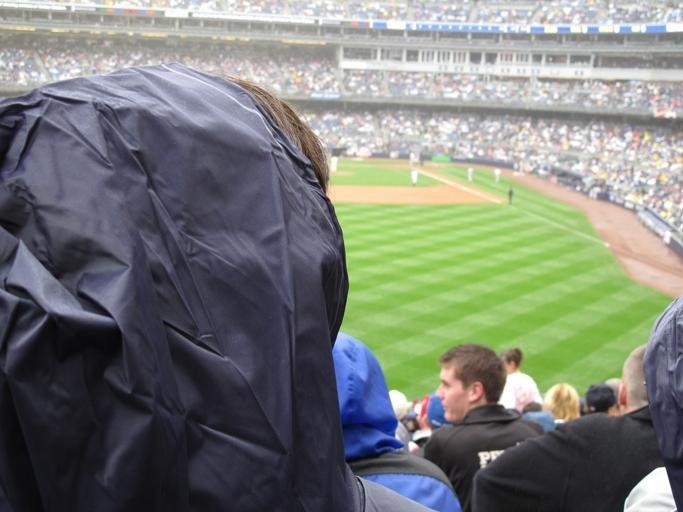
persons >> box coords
[0,0,682,242]
[0,57,429,512]
[621,292,683,511]
[330,343,683,512]
[329,326,461,511]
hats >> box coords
[585,384,614,409]
[389,387,454,429]
